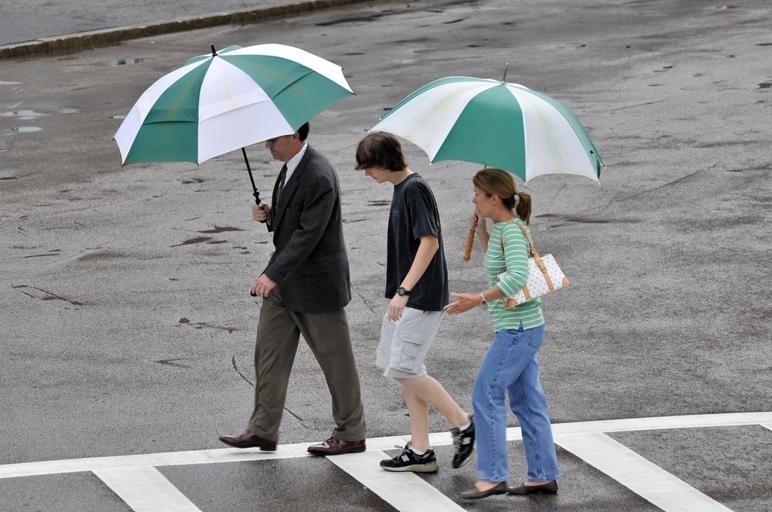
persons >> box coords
[355,131,473,472]
[219,118,366,456]
[442,169,561,499]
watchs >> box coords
[480,292,488,306]
[397,287,411,296]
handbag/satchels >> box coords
[493,217,574,311]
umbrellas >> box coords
[112,42,358,232]
[367,61,609,259]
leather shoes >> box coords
[460,476,510,501]
[306,431,368,457]
[216,425,280,454]
[507,472,562,498]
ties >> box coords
[273,164,291,218]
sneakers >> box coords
[449,412,477,470]
[378,439,442,475]
